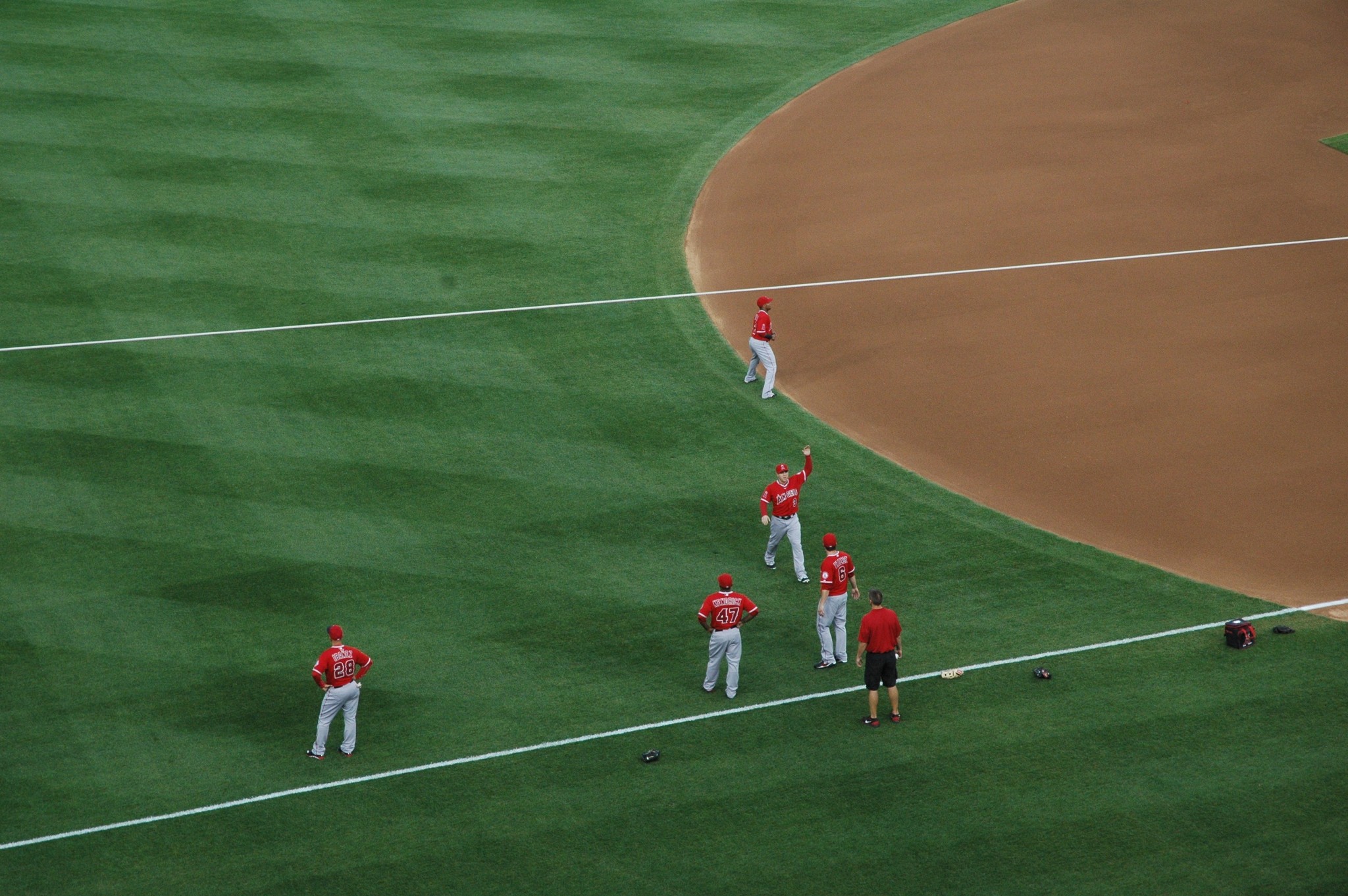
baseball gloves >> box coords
[1033,666,1051,679]
[941,668,964,679]
[641,749,660,763]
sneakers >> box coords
[860,715,880,727]
[305,750,324,760]
[337,746,352,757]
[890,709,900,723]
[834,656,847,663]
[766,561,776,570]
[798,573,810,583]
[814,659,837,668]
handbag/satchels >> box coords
[1224,618,1256,650]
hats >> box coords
[823,533,836,547]
[757,296,774,307]
[327,625,343,640]
[776,463,789,474]
[718,574,732,588]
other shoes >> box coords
[706,689,713,692]
[744,377,758,383]
[762,393,779,399]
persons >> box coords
[698,574,760,699]
[305,625,373,761]
[761,446,813,583]
[856,590,902,728]
[745,296,778,399]
[813,533,860,668]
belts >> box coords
[334,679,353,689]
[775,513,795,520]
[715,626,737,632]
